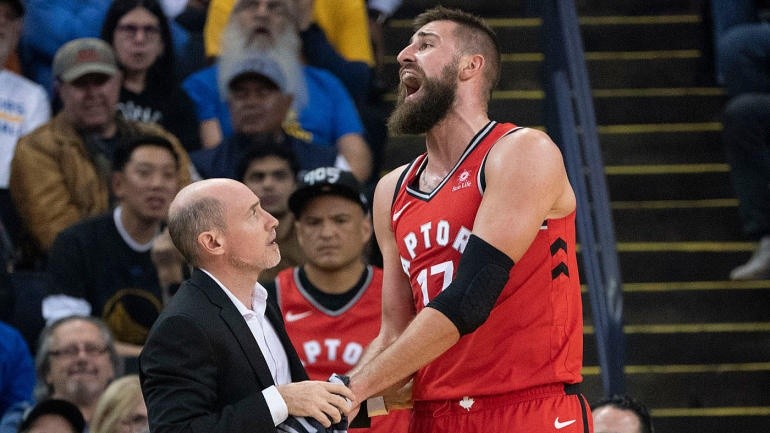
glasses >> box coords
[46,342,113,360]
[115,23,161,41]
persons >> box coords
[273,6,594,433]
[713,0,770,280]
[49,0,197,144]
[589,395,655,433]
[9,37,201,255]
[0,311,148,433]
[0,1,53,273]
[202,0,376,68]
[200,58,358,190]
[192,0,371,182]
[140,176,357,433]
[42,131,199,358]
[263,165,413,433]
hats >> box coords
[52,37,117,82]
[14,400,87,433]
[224,54,287,91]
[287,166,374,211]
